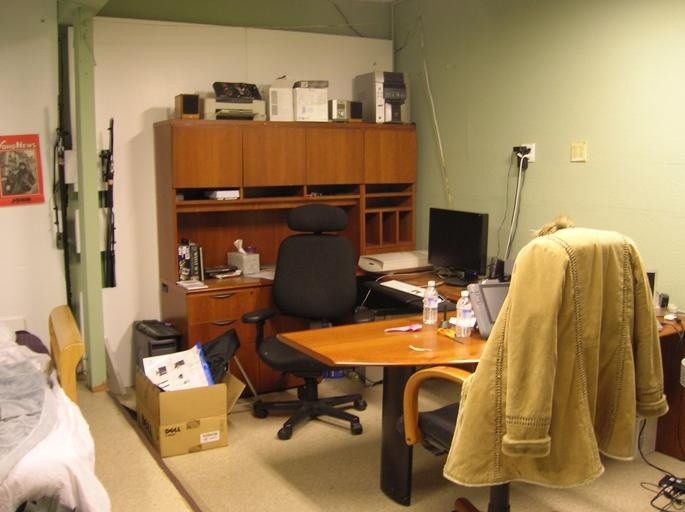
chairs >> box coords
[240,204,375,440]
[404,227,668,512]
[49,304,84,404]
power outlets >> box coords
[518,144,535,163]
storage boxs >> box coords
[135,366,246,458]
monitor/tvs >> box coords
[428,207,489,288]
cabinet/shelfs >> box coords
[153,119,419,400]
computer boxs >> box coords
[135,319,179,371]
[354,303,424,387]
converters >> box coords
[512,145,526,154]
[517,156,527,169]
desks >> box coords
[276,271,685,507]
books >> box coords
[176,238,241,291]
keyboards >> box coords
[137,320,182,340]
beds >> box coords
[0,318,112,512]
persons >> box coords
[6,161,18,194]
[15,163,35,194]
